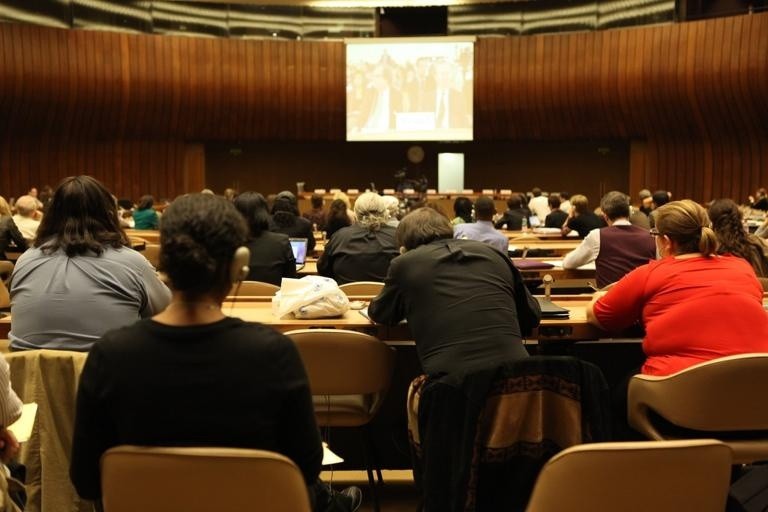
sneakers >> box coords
[341,486,362,512]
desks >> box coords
[511,256,596,293]
[508,240,582,255]
[268,191,525,223]
[221,293,646,342]
[125,227,160,242]
[297,257,318,273]
[503,228,560,239]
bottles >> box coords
[521,215,528,231]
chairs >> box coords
[525,438,734,512]
[98,445,312,511]
[5,346,90,511]
[284,328,395,490]
[230,279,281,296]
[627,352,768,464]
[419,356,605,512]
[338,281,386,295]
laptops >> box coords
[289,237,308,271]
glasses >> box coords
[650,228,660,238]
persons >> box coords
[39,185,52,204]
[7,173,173,353]
[382,195,401,228]
[268,190,315,255]
[316,192,400,287]
[12,194,40,239]
[559,191,571,213]
[748,188,767,211]
[0,351,27,512]
[367,206,543,511]
[320,198,351,240]
[234,191,296,287]
[334,192,357,225]
[223,188,239,203]
[132,194,159,231]
[528,187,551,226]
[638,188,654,214]
[346,52,472,130]
[560,190,660,289]
[561,194,605,239]
[68,190,364,511]
[544,196,569,229]
[0,195,26,280]
[29,186,43,208]
[453,197,477,223]
[586,197,766,443]
[452,196,509,253]
[302,192,328,231]
[495,195,532,230]
[647,190,669,228]
[708,197,767,280]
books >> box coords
[536,298,570,319]
